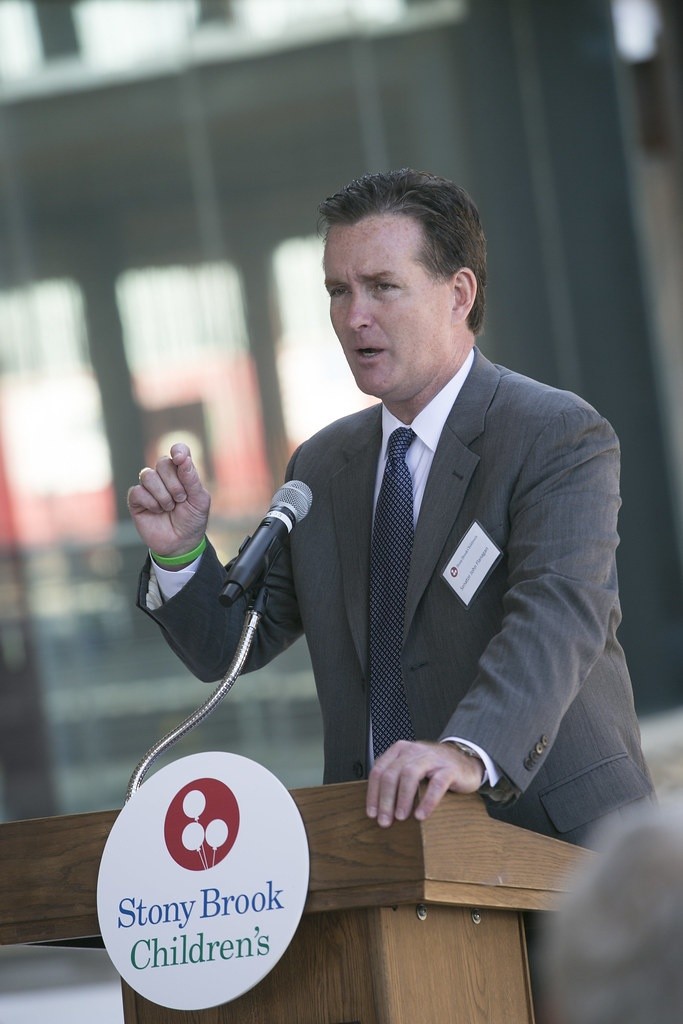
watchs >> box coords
[453,739,482,760]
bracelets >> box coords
[151,535,207,566]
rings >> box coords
[138,467,151,487]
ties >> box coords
[368,427,416,761]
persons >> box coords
[127,168,665,856]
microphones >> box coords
[217,480,313,607]
